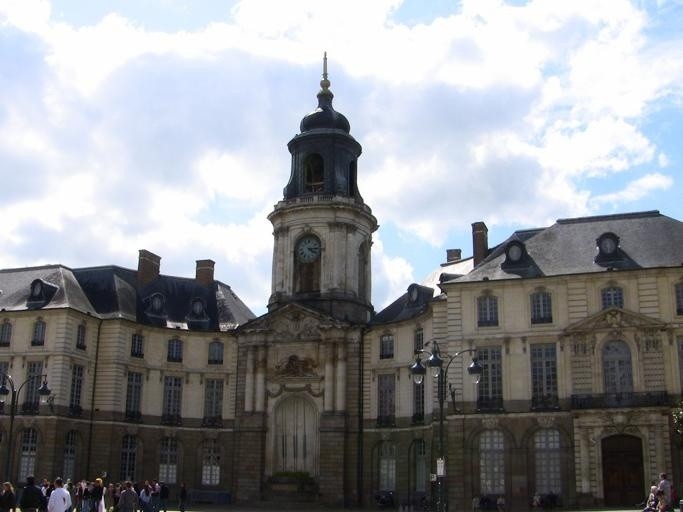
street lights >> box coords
[411,340,482,512]
[1,371,52,482]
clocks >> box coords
[294,235,320,265]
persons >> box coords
[640,472,678,512]
[532,491,543,509]
[178,481,189,512]
[496,494,506,512]
[471,494,489,512]
[547,491,558,506]
[0,475,170,512]
